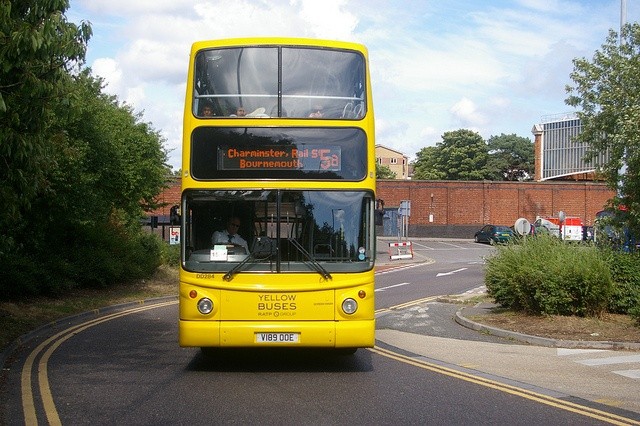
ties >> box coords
[227,235,235,242]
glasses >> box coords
[314,110,323,113]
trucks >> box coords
[531,217,584,245]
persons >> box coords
[306,104,326,118]
[211,218,250,255]
[229,105,249,117]
[199,101,216,117]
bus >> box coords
[170,36,387,359]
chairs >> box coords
[314,244,332,261]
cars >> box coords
[584,226,593,243]
[474,223,514,246]
